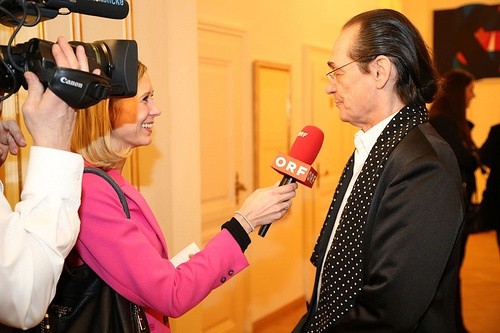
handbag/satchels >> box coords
[41,167,151,332]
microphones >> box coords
[257,126,324,237]
[47,0,129,19]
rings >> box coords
[284,202,290,210]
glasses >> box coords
[325,55,374,85]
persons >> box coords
[66,61,298,333]
[429,69,481,196]
[0,35,101,331]
[477,122,500,246]
[292,9,472,333]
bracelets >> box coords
[234,211,254,232]
